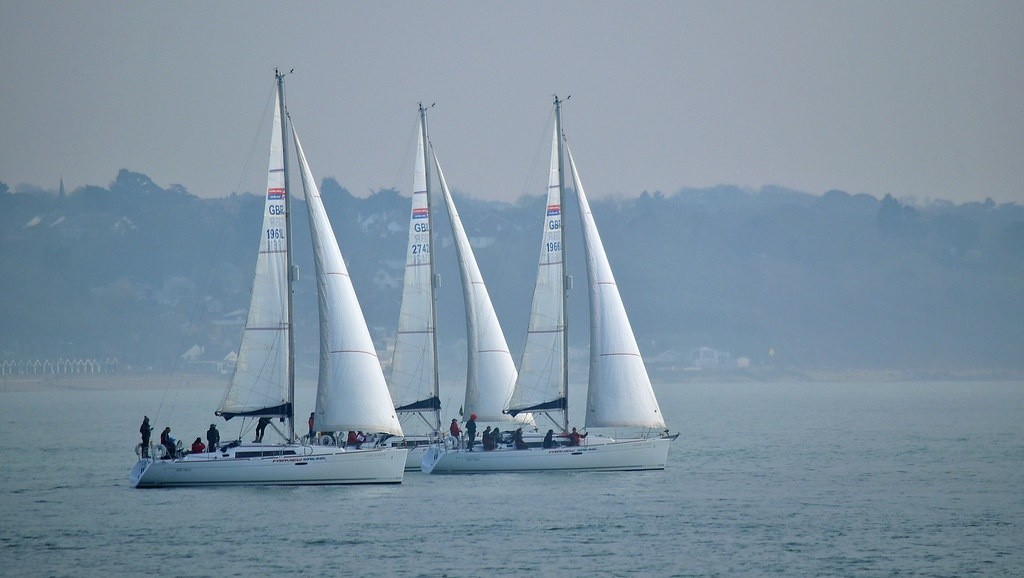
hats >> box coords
[144,416,149,422]
[452,419,457,422]
[470,414,478,420]
[166,427,171,431]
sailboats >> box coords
[383,98,542,473]
[420,94,681,475]
[130,66,412,488]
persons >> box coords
[348,430,367,450]
[191,438,206,453]
[514,428,529,450]
[482,426,502,451]
[139,416,153,458]
[252,416,272,443]
[207,423,220,453]
[308,412,315,433]
[465,414,478,452]
[560,427,588,448]
[450,419,463,449]
[161,426,176,459]
[543,430,557,448]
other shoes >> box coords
[466,447,469,449]
[252,439,262,443]
[469,450,473,452]
